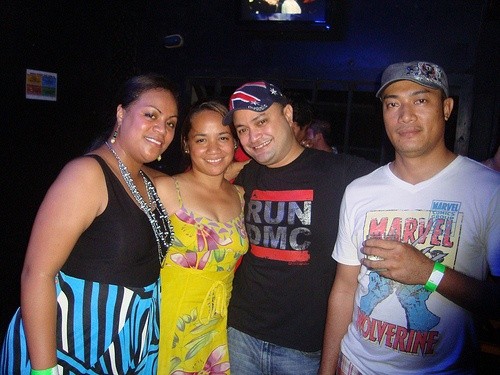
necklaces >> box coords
[103,138,176,269]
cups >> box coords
[366,234,399,272]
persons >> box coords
[305,118,339,154]
[152,100,250,375]
[233,101,316,162]
[315,62,500,375]
[0,73,180,375]
[220,79,357,375]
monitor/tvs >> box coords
[231,0,337,33]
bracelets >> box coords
[422,260,446,293]
[30,364,64,375]
